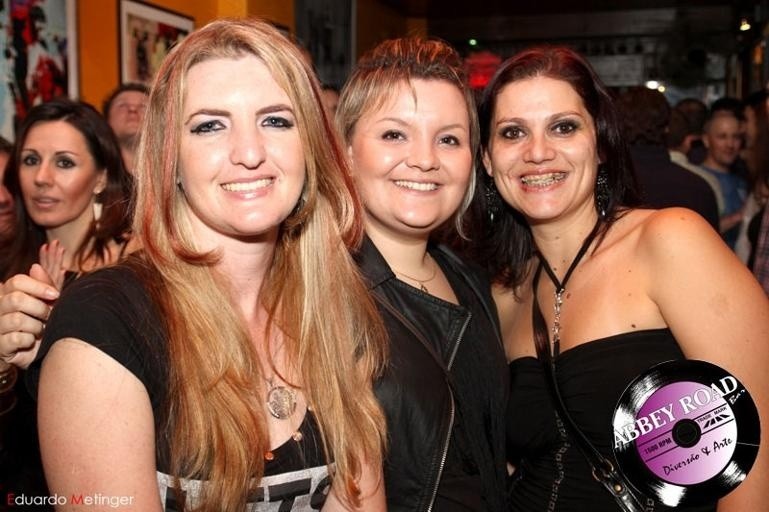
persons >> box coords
[0,11,769,512]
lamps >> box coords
[737,11,752,31]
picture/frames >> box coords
[0,0,80,154]
[118,0,195,93]
